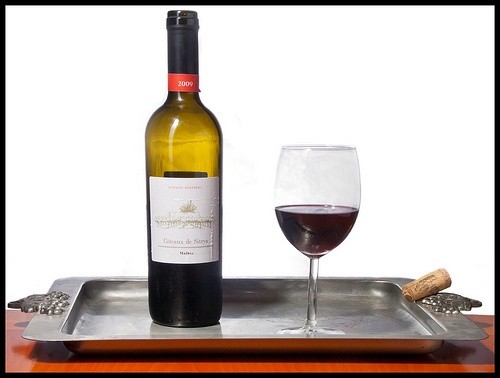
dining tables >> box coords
[4,308,495,375]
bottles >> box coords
[144,10,222,328]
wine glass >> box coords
[275,145,360,336]
